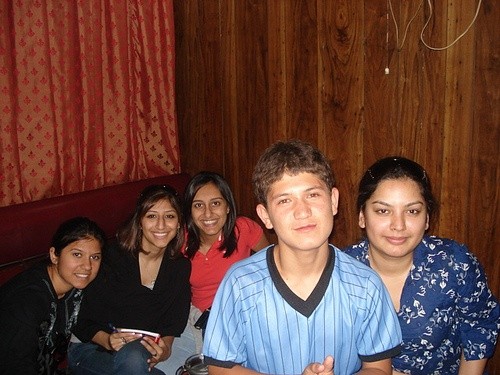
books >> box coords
[113,326,161,345]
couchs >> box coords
[0,173,194,369]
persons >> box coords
[201,140,404,375]
[181,170,270,313]
[65,183,194,375]
[0,214,107,375]
[337,156,500,375]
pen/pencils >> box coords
[110,325,117,333]
[154,336,160,343]
